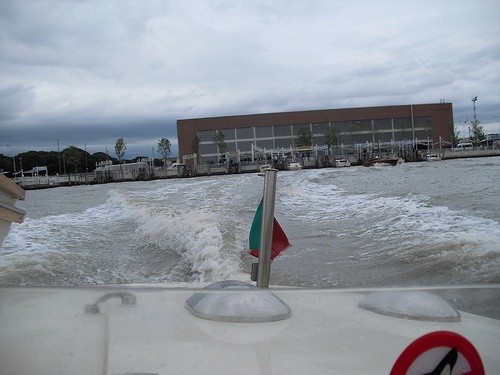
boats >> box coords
[257,136,444,174]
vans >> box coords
[454,142,473,151]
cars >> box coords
[168,162,184,171]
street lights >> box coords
[6,144,16,180]
[472,96,477,122]
